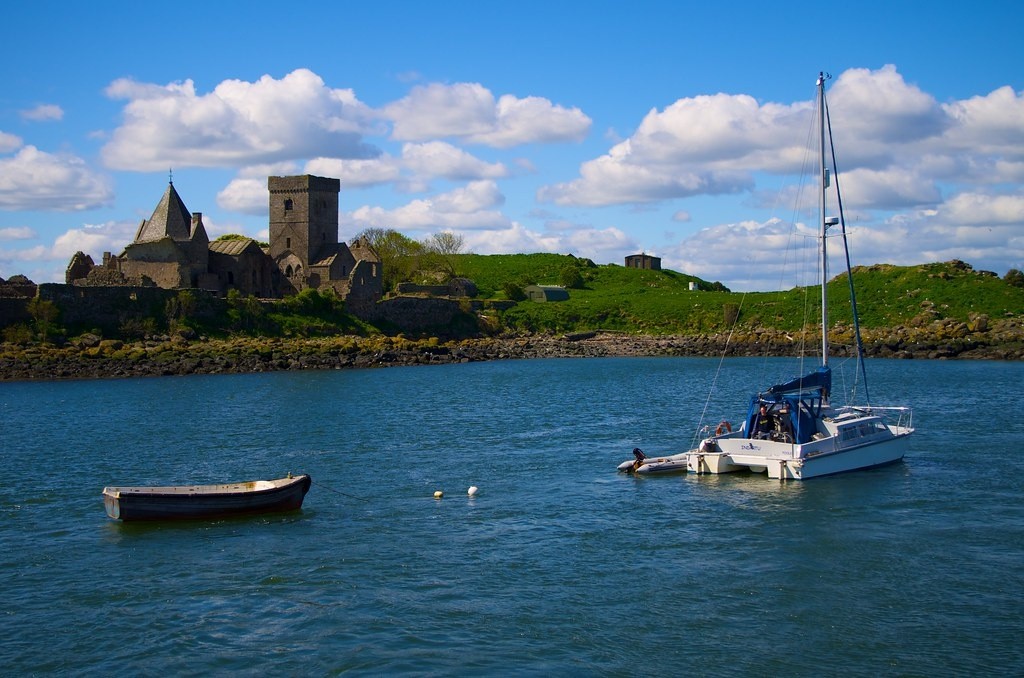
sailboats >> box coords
[686,71,915,481]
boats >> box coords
[616,451,686,476]
[103,473,313,519]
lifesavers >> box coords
[715,420,732,437]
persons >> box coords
[751,405,774,440]
